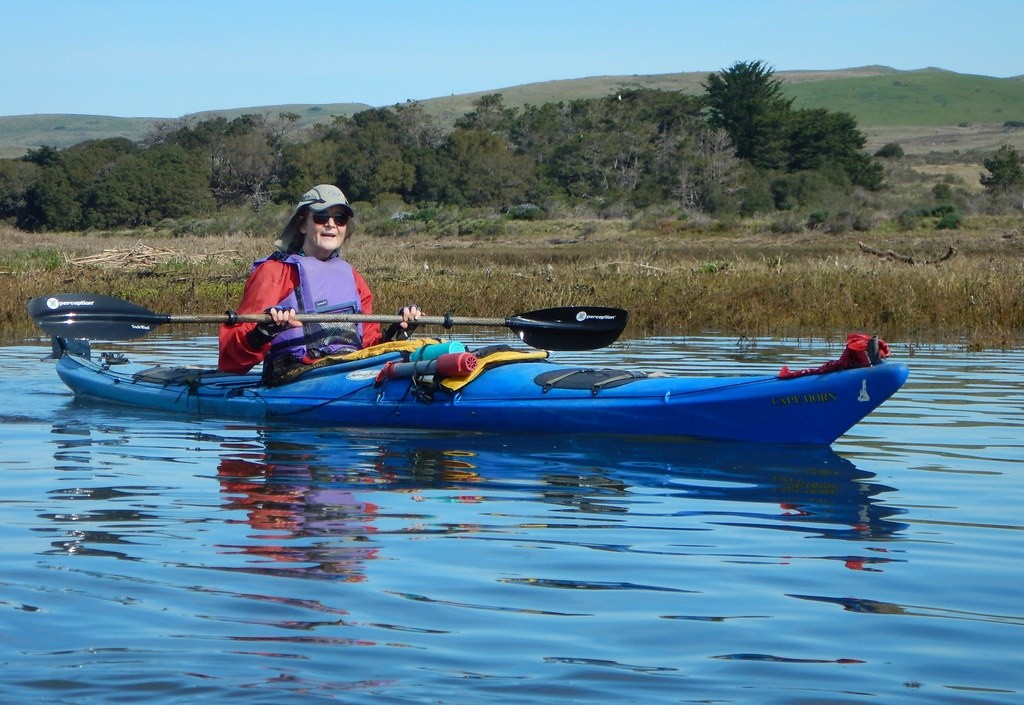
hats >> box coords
[304,184,354,218]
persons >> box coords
[217,183,428,375]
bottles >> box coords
[409,340,466,362]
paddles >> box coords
[28,294,628,352]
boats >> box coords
[41,334,910,464]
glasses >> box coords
[313,210,348,226]
